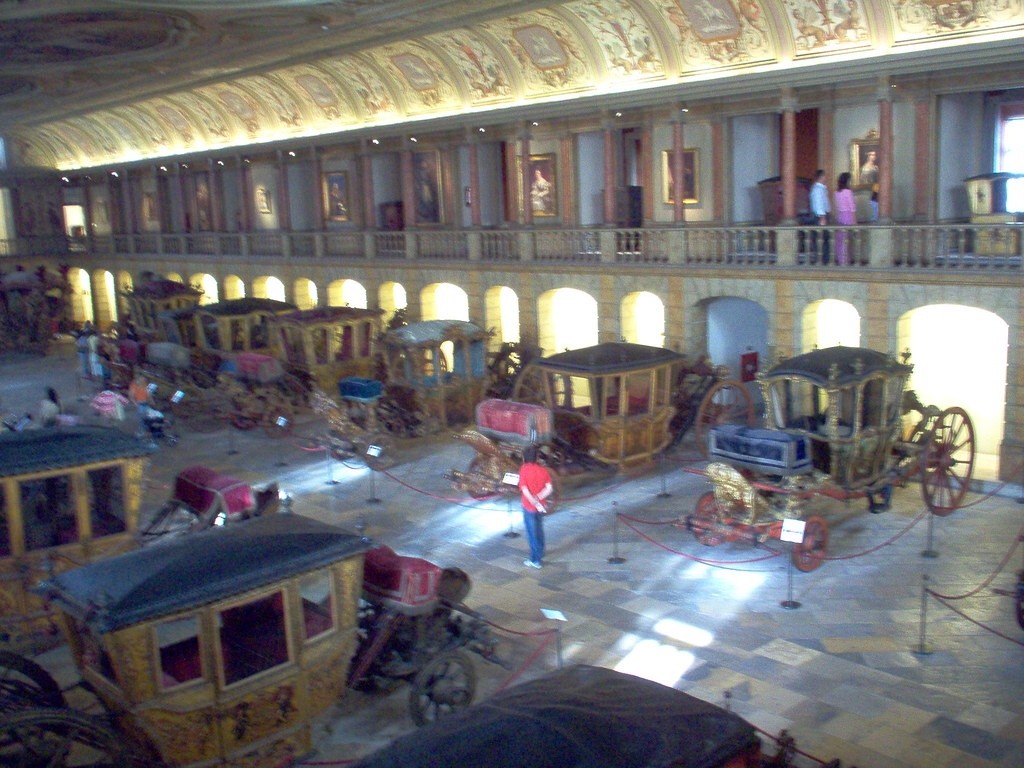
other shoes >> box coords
[524,559,543,570]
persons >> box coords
[127,374,156,439]
[868,183,879,224]
[808,169,831,266]
[39,387,62,429]
[519,445,554,570]
[89,376,129,422]
[73,318,142,383]
[832,172,857,266]
